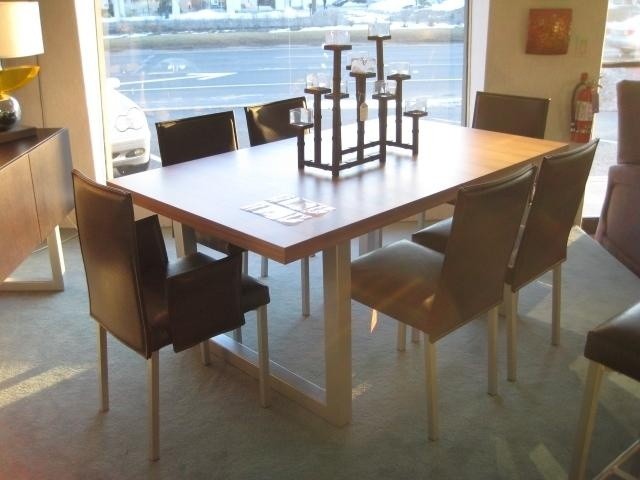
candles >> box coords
[289,21,427,130]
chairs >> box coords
[415,91,552,234]
[594,78,639,280]
[409,135,601,383]
[349,162,542,443]
[571,298,640,480]
[156,109,311,347]
[243,95,314,147]
[71,168,272,464]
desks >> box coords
[106,114,570,427]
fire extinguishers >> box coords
[570,73,603,143]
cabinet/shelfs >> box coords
[1,126,72,297]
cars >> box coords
[605,15,640,54]
[105,77,151,175]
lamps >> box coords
[1,2,44,139]
